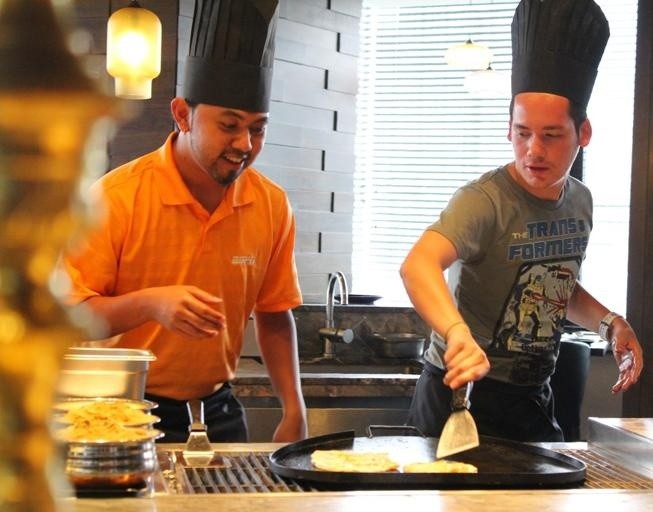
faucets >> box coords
[317,271,354,361]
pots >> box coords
[52,415,161,429]
[267,425,587,490]
[54,430,165,489]
[51,396,160,415]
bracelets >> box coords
[599,312,623,342]
[444,321,469,345]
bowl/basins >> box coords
[379,333,427,357]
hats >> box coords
[183,0,279,112]
[511,0,610,109]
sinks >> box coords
[298,357,426,379]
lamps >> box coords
[445,1,495,71]
[105,0,161,100]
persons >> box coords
[47,0,308,447]
[399,0,643,444]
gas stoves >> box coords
[172,450,652,494]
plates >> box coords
[334,295,381,304]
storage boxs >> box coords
[59,347,157,402]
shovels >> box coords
[182,399,214,466]
[436,381,480,459]
